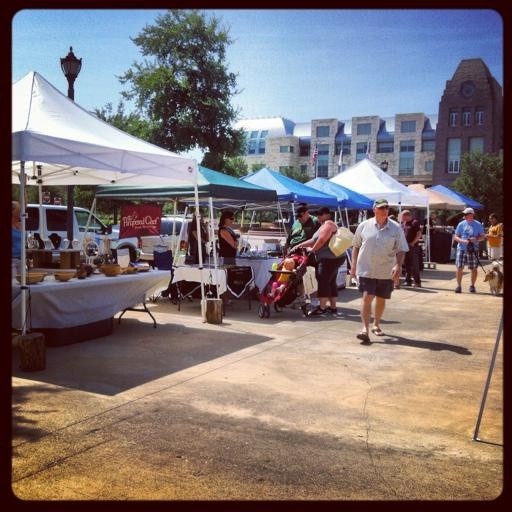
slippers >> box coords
[356,329,370,343]
[371,325,384,336]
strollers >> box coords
[258,246,311,318]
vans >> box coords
[25,204,139,263]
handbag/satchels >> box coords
[328,227,355,257]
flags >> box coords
[312,142,318,165]
[337,149,344,166]
[366,141,371,160]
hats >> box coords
[295,206,308,213]
[374,199,389,208]
[462,207,474,214]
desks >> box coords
[12,270,171,330]
[172,257,286,316]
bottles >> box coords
[27,232,34,248]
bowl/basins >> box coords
[101,264,120,276]
[54,272,75,282]
[16,272,47,284]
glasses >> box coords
[379,206,389,210]
[463,213,471,216]
[227,217,234,220]
[296,213,305,218]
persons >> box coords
[400,209,421,287]
[349,198,409,341]
[267,258,296,298]
[454,207,486,293]
[217,210,240,312]
[389,214,408,290]
[283,206,321,267]
[187,207,209,264]
[12,201,26,279]
[422,210,465,235]
[290,207,346,318]
[485,212,503,264]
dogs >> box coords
[484,265,504,296]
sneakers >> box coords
[455,287,461,293]
[308,306,329,319]
[320,307,338,317]
[469,285,475,292]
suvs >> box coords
[139,214,251,267]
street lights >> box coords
[59,45,83,245]
[380,160,388,171]
